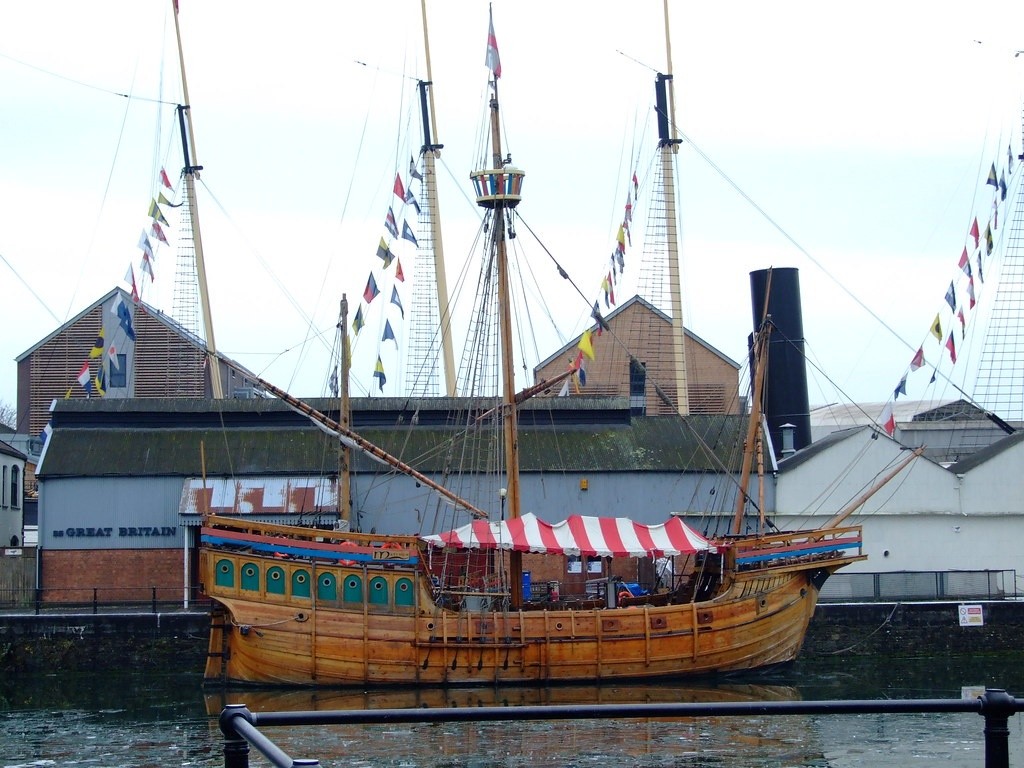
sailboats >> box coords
[172,0,925,683]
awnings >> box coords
[178,476,341,609]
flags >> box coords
[40,168,184,442]
[484,20,501,79]
[879,140,1024,435]
[329,157,422,398]
[560,172,638,397]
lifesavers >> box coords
[337,542,359,566]
[271,537,291,557]
[380,543,403,568]
[618,592,633,606]
